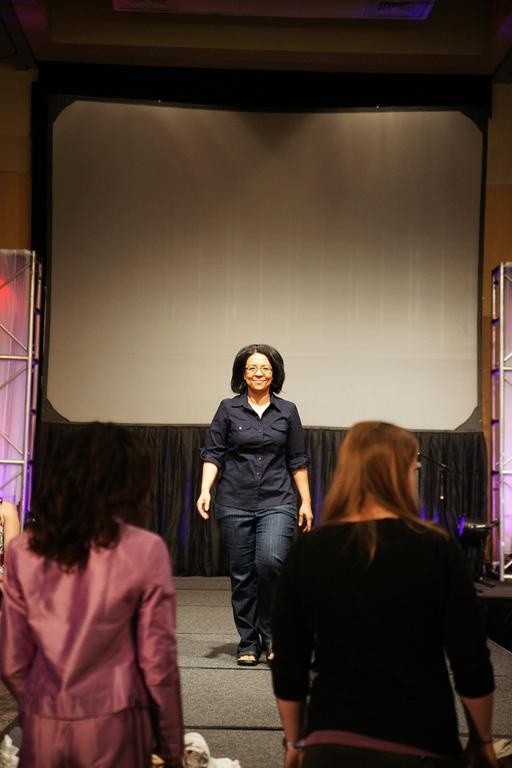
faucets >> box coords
[237,654,260,666]
[266,650,276,662]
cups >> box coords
[245,365,272,373]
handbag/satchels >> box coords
[468,738,488,744]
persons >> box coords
[281,739,301,749]
[193,342,316,666]
[0,420,188,767]
[0,498,21,768]
[267,421,506,768]
